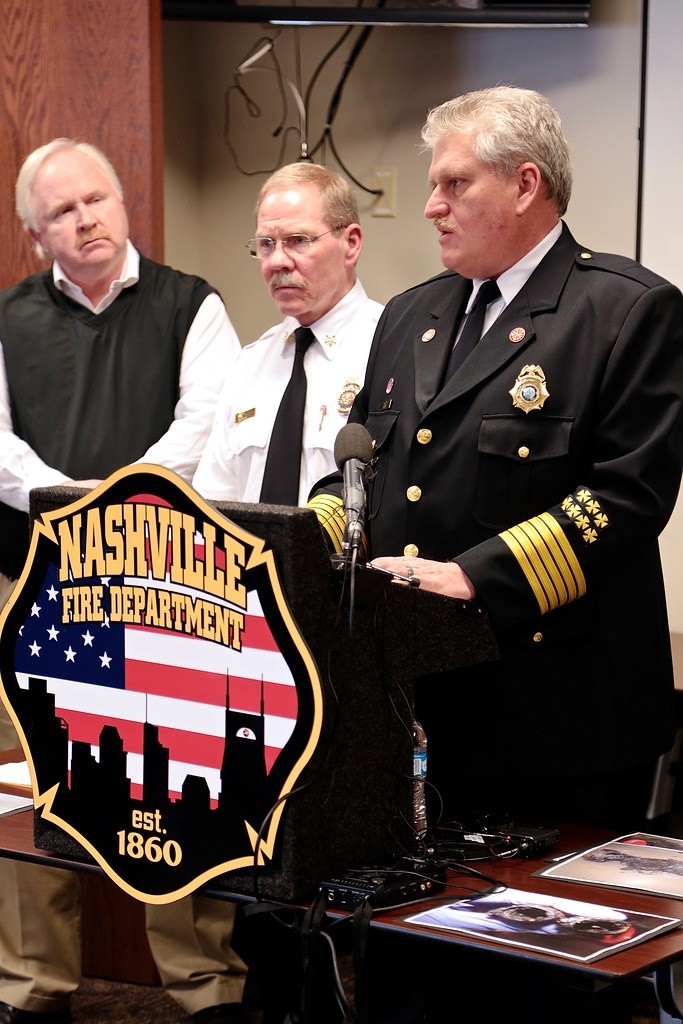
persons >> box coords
[0,138,245,1024]
[191,163,385,509]
[303,86,683,833]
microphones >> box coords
[334,423,373,548]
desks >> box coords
[0,747,683,1024]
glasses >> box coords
[245,226,338,259]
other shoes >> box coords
[191,1002,248,1024]
[0,1002,76,1024]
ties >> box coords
[442,281,501,392]
[259,326,316,507]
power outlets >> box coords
[372,165,396,217]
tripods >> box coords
[336,528,421,588]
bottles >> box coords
[413,719,428,840]
